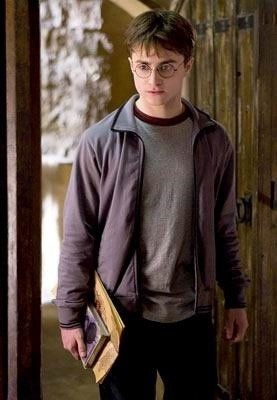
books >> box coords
[90,269,124,385]
[80,302,110,369]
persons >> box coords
[52,7,251,400]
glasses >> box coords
[130,57,186,78]
